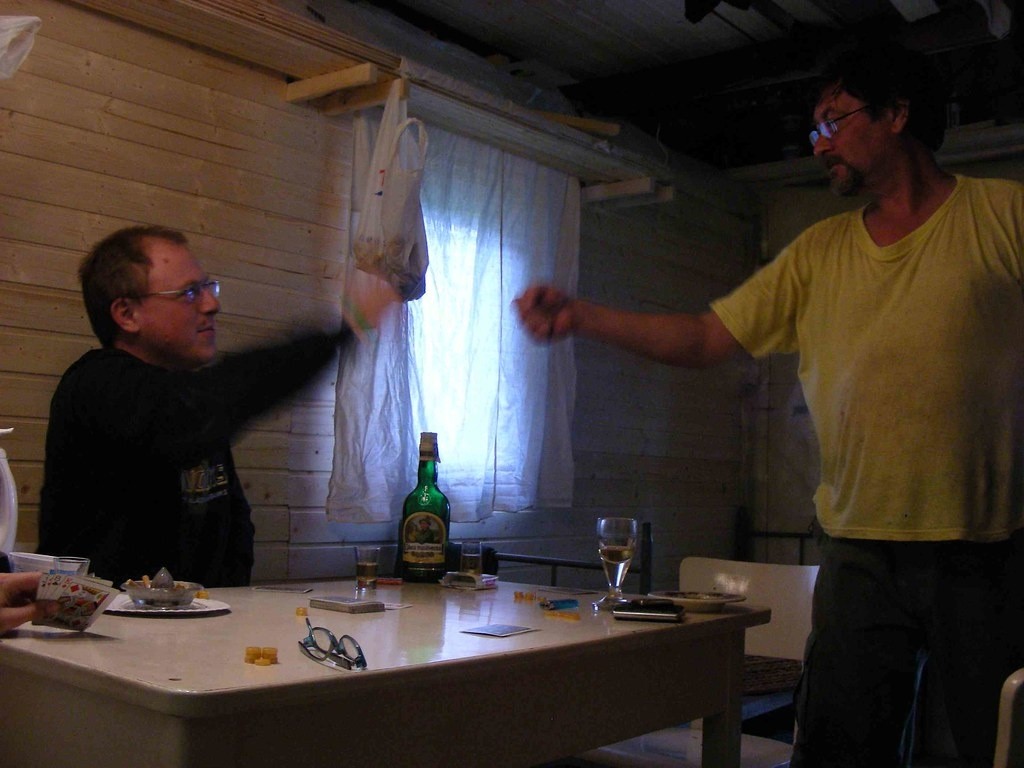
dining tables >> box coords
[1,573,773,768]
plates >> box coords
[121,581,204,610]
[649,593,746,613]
[105,592,232,615]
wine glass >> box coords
[591,518,638,614]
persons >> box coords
[0,420,61,641]
[516,40,1024,767]
[34,227,404,590]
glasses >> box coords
[809,99,891,148]
[298,615,367,671]
[126,280,221,303]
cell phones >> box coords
[613,604,685,621]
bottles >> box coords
[399,433,451,584]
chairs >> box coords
[992,667,1024,768]
[573,556,821,768]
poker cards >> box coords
[310,596,413,614]
[461,623,541,638]
[252,585,314,594]
[32,572,120,629]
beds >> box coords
[449,512,821,722]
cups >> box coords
[461,542,482,582]
[355,544,380,591]
[54,557,90,575]
[9,552,83,573]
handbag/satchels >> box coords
[354,77,428,300]
